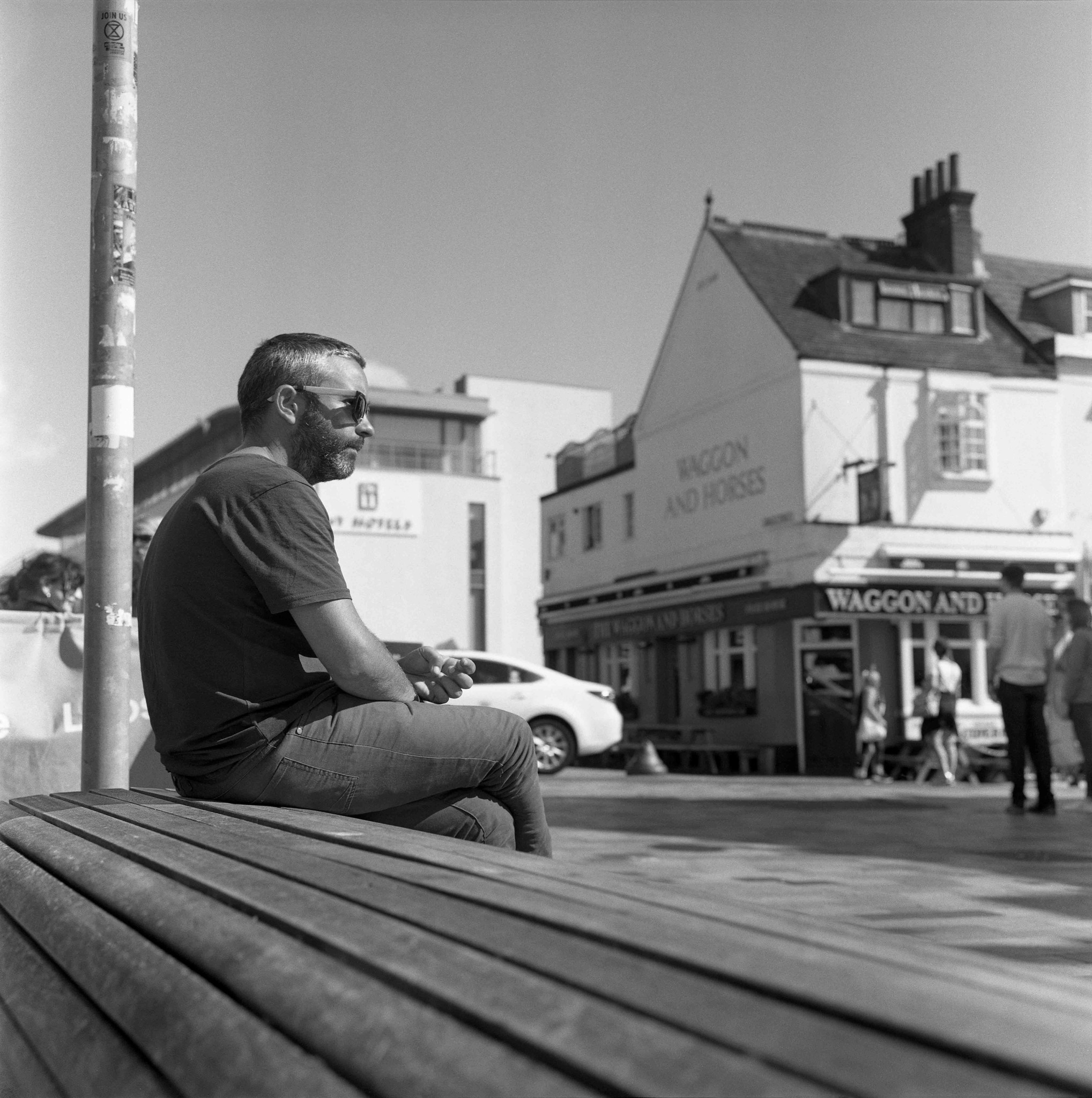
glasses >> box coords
[267,383,369,424]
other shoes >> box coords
[1029,803,1054,815]
[1008,803,1024,814]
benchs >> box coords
[0,787,1092,1098]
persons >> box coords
[138,332,552,860]
[925,639,963,784]
[855,665,893,783]
[1058,598,1092,800]
[986,564,1058,816]
[915,673,980,787]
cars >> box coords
[435,649,626,775]
